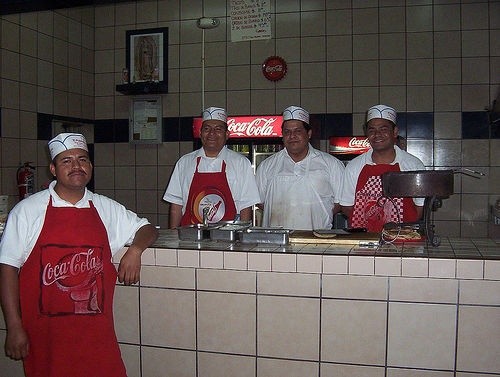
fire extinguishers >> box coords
[16,162,36,202]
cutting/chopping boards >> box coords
[290,229,381,246]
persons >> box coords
[255,106,345,232]
[339,105,428,234]
[0,133,159,377]
[162,107,261,231]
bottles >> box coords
[207,201,223,221]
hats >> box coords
[367,104,397,125]
[203,107,227,124]
[283,106,310,125]
[48,132,89,161]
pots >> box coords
[381,169,485,197]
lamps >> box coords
[196,17,221,29]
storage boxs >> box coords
[173,224,210,240]
[207,220,252,243]
[236,226,290,245]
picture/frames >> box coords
[125,27,168,84]
